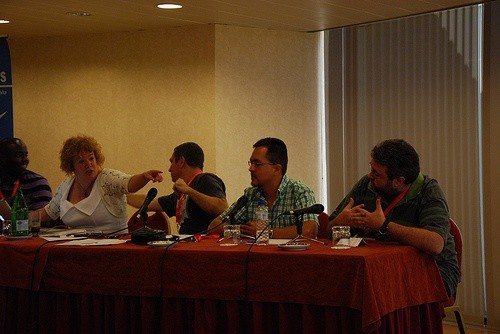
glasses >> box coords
[371,166,387,179]
[248,161,273,167]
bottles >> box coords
[11,187,29,236]
[256,201,269,246]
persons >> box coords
[325,138,461,308]
[207,138,319,240]
[0,138,53,227]
[127,142,228,236]
[28,136,163,237]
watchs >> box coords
[378,219,389,237]
[269,228,273,239]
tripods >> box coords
[290,215,328,245]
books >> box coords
[268,238,292,244]
[40,237,131,246]
[166,234,193,241]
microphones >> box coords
[137,188,157,220]
[284,204,324,215]
[222,196,248,222]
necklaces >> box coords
[75,176,94,196]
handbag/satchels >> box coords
[130,227,167,243]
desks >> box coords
[0,228,446,334]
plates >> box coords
[147,240,175,246]
[278,243,310,250]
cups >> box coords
[30,210,42,237]
[223,225,240,244]
[332,226,350,249]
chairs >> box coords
[127,209,171,235]
